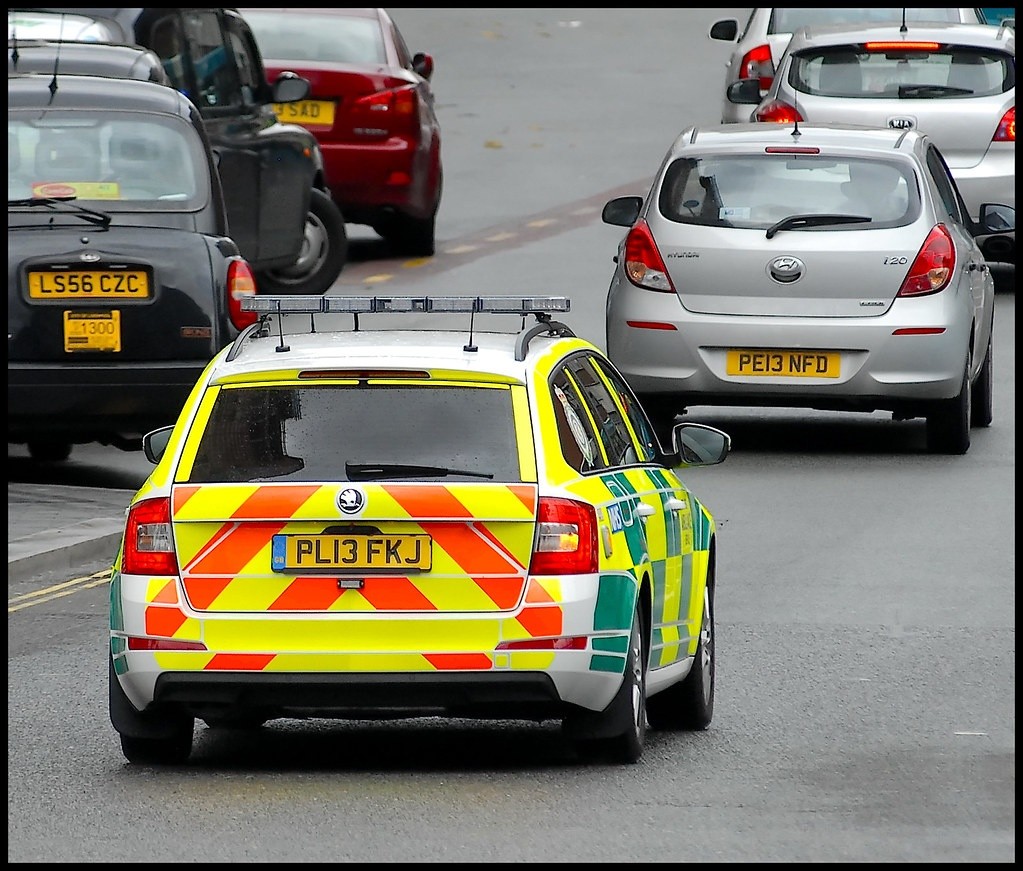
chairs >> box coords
[946,55,990,96]
[819,54,863,93]
[836,163,907,222]
[688,160,762,222]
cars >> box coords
[707,8,1015,124]
[0,6,444,293]
[726,16,1015,265]
[603,118,1015,458]
[6,70,261,463]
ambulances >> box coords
[106,291,731,765]
